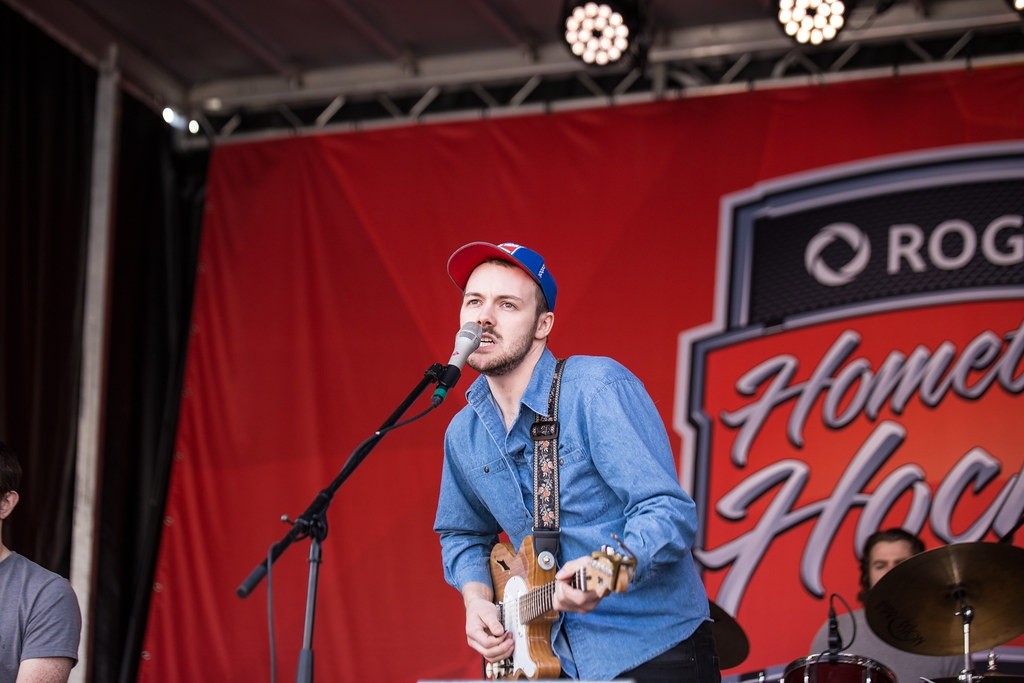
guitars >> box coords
[484,534,639,682]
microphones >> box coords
[828,608,842,653]
[431,321,482,406]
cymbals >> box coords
[864,541,1023,657]
[707,598,749,670]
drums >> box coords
[784,652,896,683]
[972,654,1024,683]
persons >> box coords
[0,440,81,683]
[434,241,722,683]
[806,527,975,683]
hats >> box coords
[447,242,558,314]
